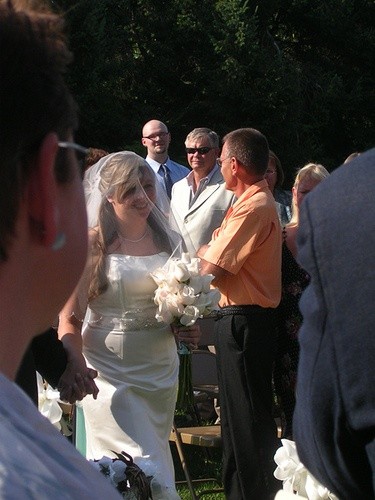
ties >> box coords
[160,164,173,200]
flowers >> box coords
[38,384,62,432]
[90,456,128,487]
[272,439,338,500]
[151,253,222,414]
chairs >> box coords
[169,350,224,500]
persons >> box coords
[169,127,236,256]
[142,120,190,207]
[15,328,98,414]
[264,151,294,224]
[57,152,202,500]
[292,148,375,500]
[199,128,282,500]
[0,0,124,500]
[275,163,329,437]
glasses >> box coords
[264,169,275,174]
[215,156,244,167]
[58,142,92,180]
[185,147,216,154]
[143,133,168,140]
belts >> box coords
[219,307,269,316]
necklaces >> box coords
[117,224,148,243]
